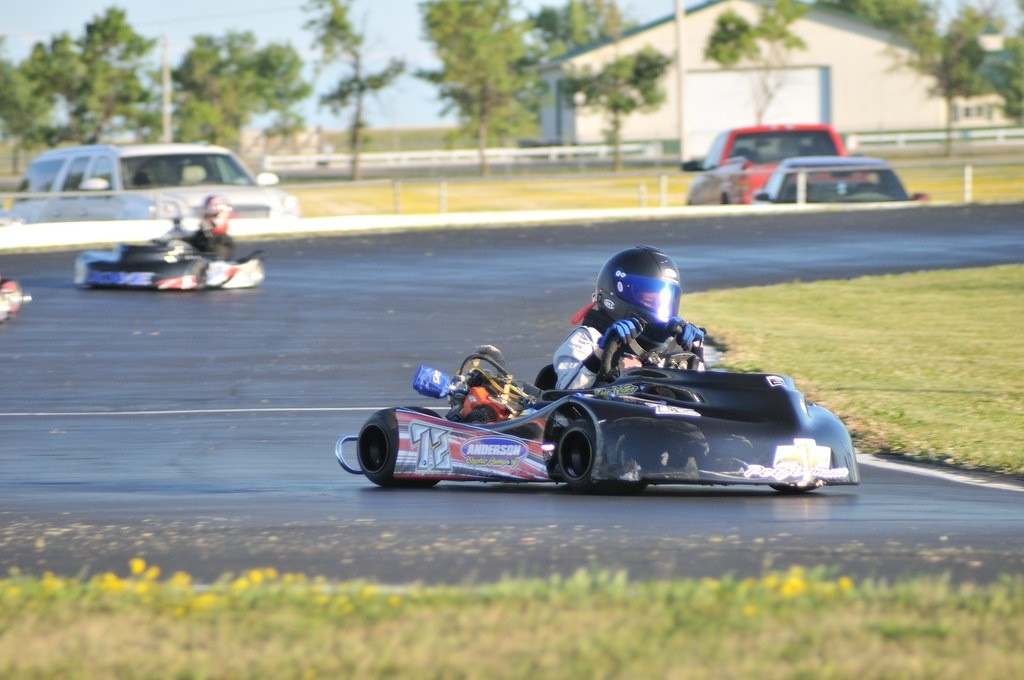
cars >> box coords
[750,157,929,207]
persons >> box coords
[553,247,705,390]
[189,196,235,262]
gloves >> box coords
[597,316,648,349]
[668,316,705,352]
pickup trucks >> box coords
[680,123,849,206]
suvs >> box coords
[9,139,300,222]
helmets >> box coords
[595,244,681,321]
[201,197,232,235]
